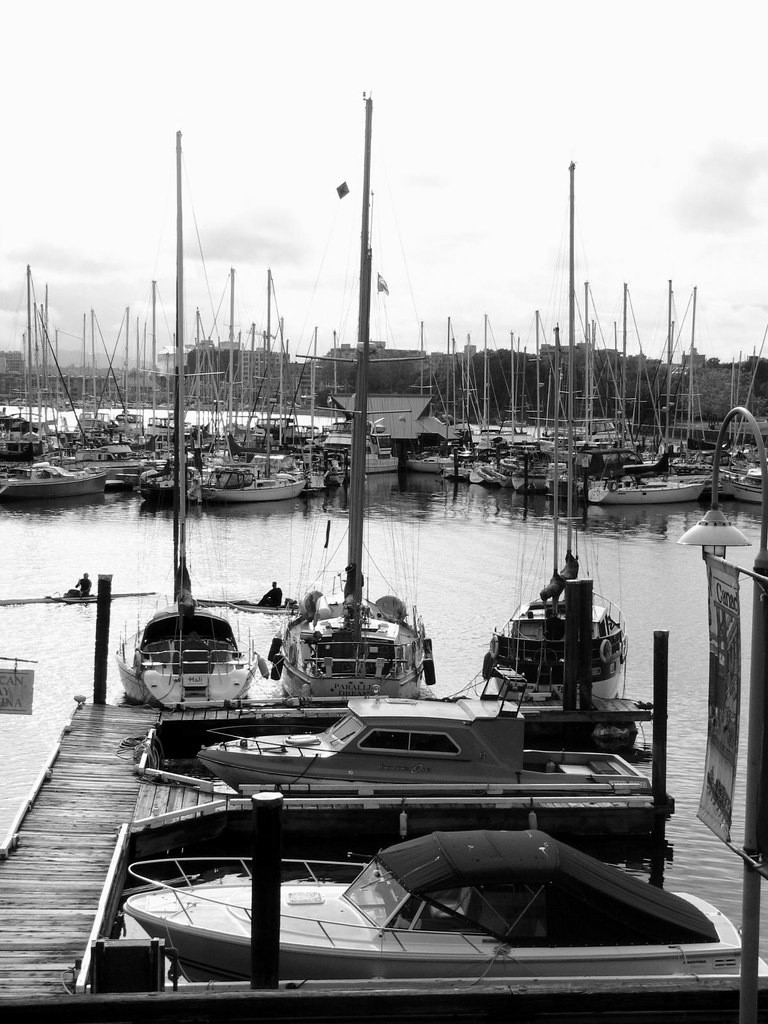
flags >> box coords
[377,272,389,296]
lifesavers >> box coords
[489,634,498,659]
[608,481,618,492]
[285,734,320,745]
[599,639,612,664]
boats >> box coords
[191,694,654,797]
[122,817,742,978]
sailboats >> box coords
[0,261,768,509]
[476,159,630,705]
[113,129,270,707]
[268,85,436,698]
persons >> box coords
[75,573,95,597]
[256,582,282,607]
